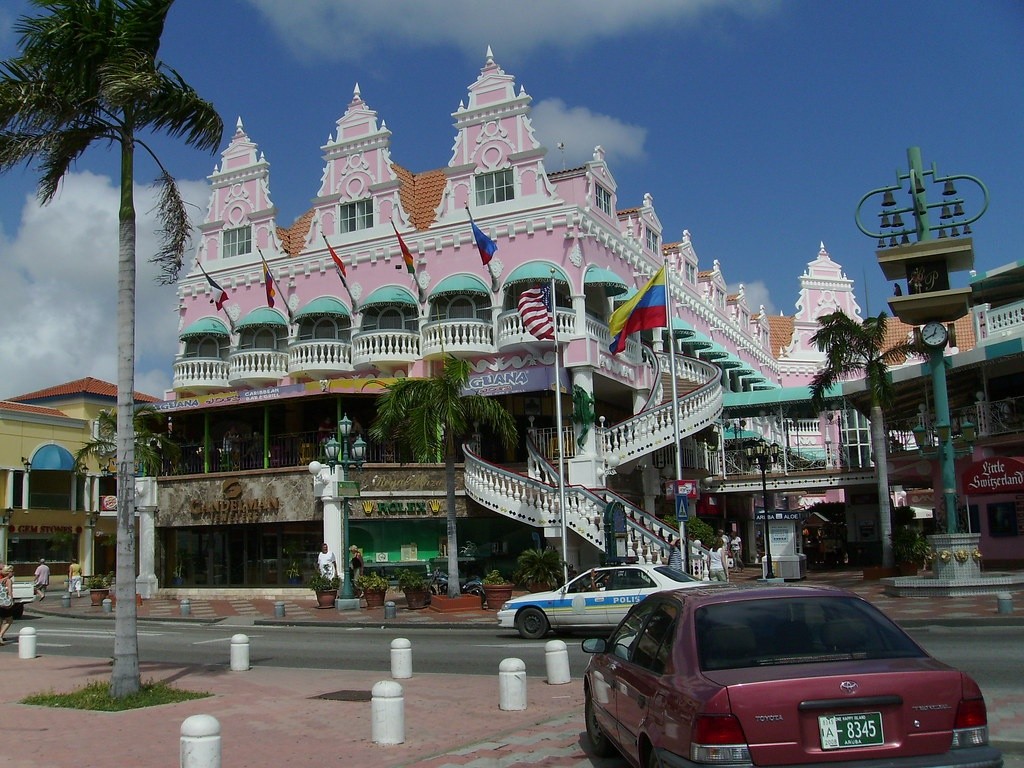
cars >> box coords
[580,581,1005,768]
[495,556,728,640]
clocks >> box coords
[921,322,947,347]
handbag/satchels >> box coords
[352,557,362,569]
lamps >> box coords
[90,510,100,525]
[3,507,14,523]
[99,463,108,479]
[21,457,31,473]
[77,462,89,477]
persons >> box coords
[591,568,610,592]
[0,565,15,644]
[349,545,364,580]
[317,543,337,581]
[669,538,682,570]
[69,559,82,598]
[707,529,745,582]
[34,559,50,601]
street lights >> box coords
[852,146,992,537]
[322,412,368,599]
[742,435,780,580]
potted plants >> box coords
[482,569,515,609]
[393,568,428,609]
[356,571,389,606]
[308,575,340,608]
[87,571,115,606]
[516,547,563,592]
[889,527,930,576]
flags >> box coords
[328,246,346,287]
[518,283,554,341]
[263,261,275,308]
[608,262,668,356]
[396,231,415,274]
[470,219,498,265]
[205,273,229,311]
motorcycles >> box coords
[425,568,487,606]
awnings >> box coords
[179,264,567,343]
[29,444,76,470]
[584,267,629,299]
[724,427,816,461]
[717,382,857,419]
[614,288,776,390]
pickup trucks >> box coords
[11,581,38,619]
